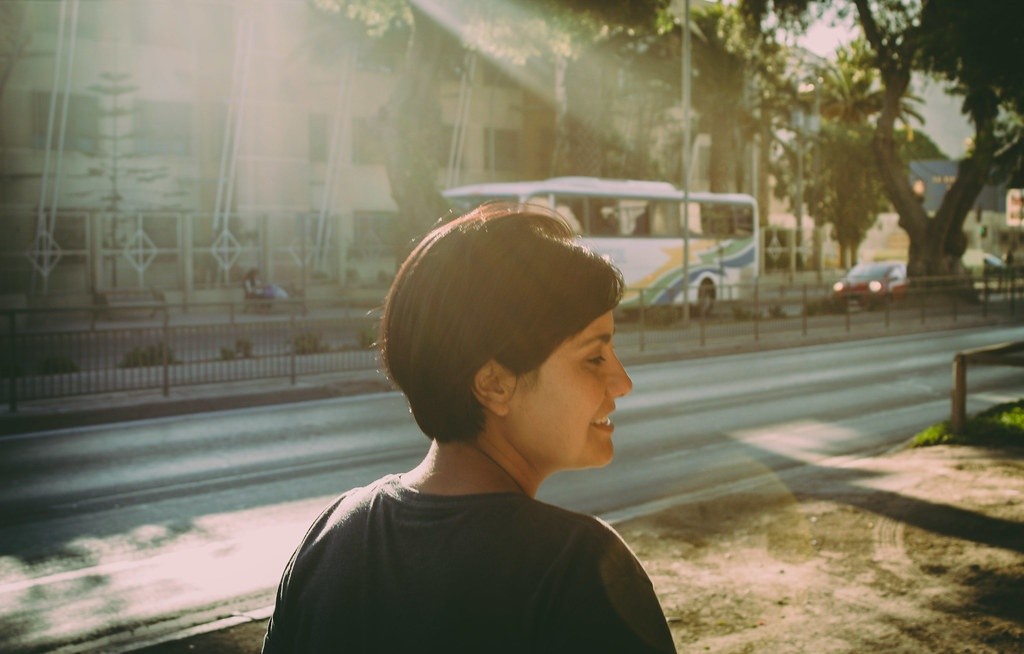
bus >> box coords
[441,176,764,316]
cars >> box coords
[834,260,908,307]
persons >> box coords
[258,201,674,654]
